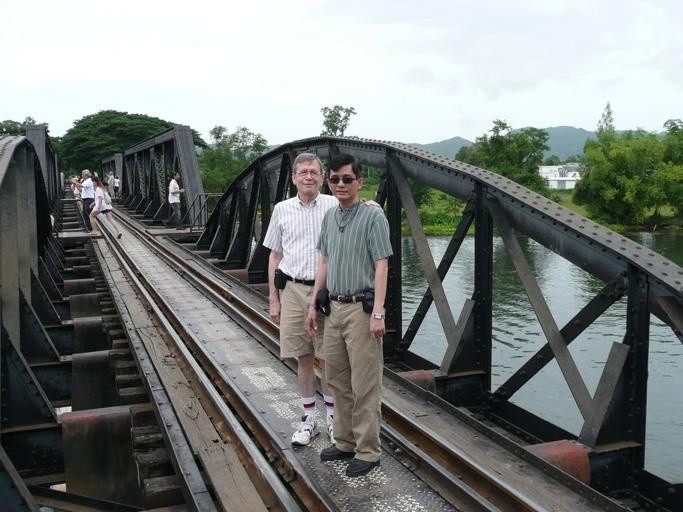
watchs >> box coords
[371,312,386,319]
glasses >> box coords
[327,174,359,184]
[293,170,325,178]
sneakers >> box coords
[82,229,97,235]
[117,232,122,238]
[291,415,320,446]
[325,415,336,444]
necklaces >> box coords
[333,199,359,232]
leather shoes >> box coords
[345,458,380,477]
[320,445,356,461]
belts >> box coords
[329,292,364,304]
[284,275,314,285]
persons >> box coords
[69,169,120,231]
[302,153,394,477]
[87,176,121,238]
[163,171,187,231]
[261,154,382,445]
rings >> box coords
[375,334,379,338]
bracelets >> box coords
[306,303,318,310]
